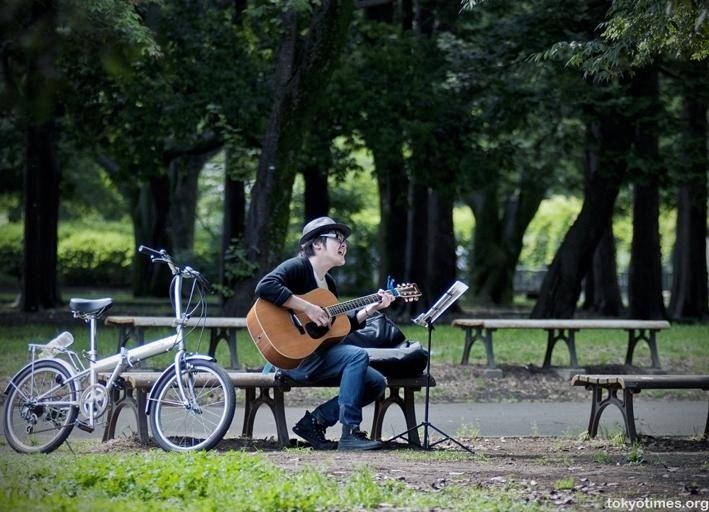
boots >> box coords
[292,410,335,450]
[338,424,383,450]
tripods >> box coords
[383,316,477,455]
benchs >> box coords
[100,315,438,444]
[451,316,709,447]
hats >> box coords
[297,216,352,249]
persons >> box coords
[253,217,396,453]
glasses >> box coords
[319,233,350,248]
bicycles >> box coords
[2,245,236,456]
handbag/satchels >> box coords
[342,308,428,379]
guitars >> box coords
[246,283,422,370]
